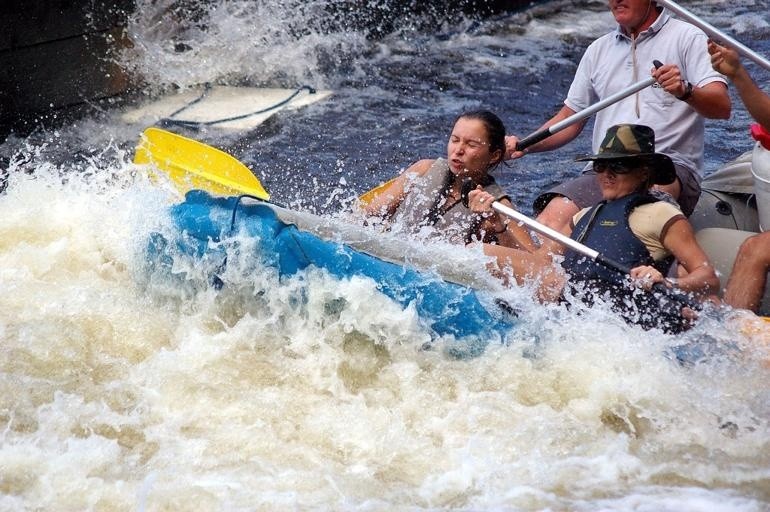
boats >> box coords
[138,191,738,368]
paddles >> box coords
[134,129,269,200]
[461,178,770,366]
[354,59,663,218]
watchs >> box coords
[664,277,679,289]
[675,79,692,101]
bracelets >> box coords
[496,223,508,233]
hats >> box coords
[574,123,676,184]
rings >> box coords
[479,198,484,203]
[646,273,651,279]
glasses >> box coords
[592,162,635,175]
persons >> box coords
[706,38,770,315]
[504,0,732,250]
[340,109,538,254]
[466,123,722,320]
